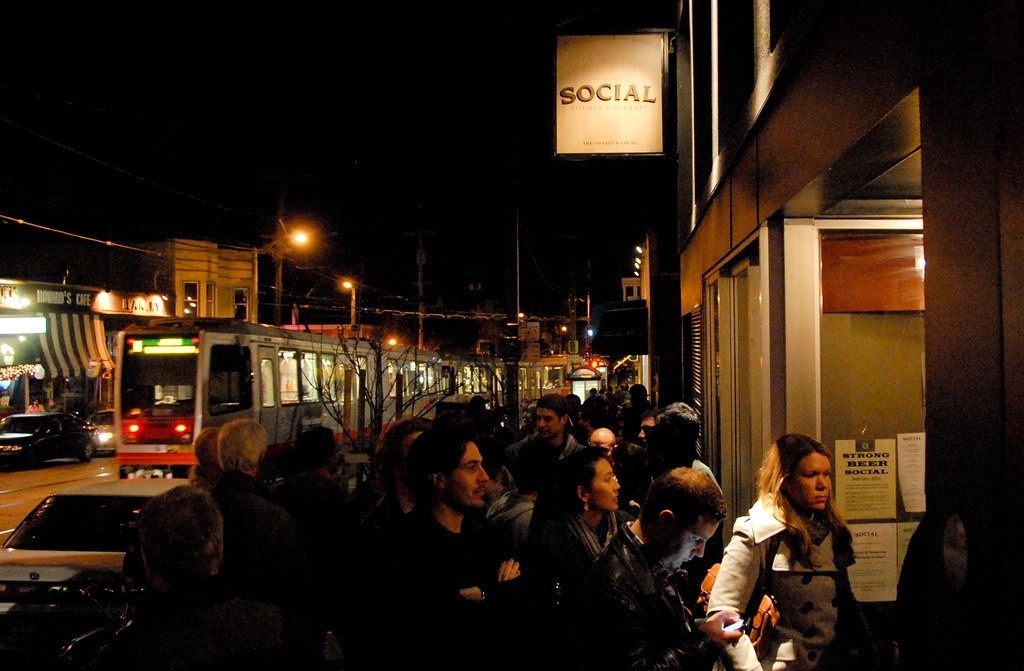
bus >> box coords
[111,317,574,481]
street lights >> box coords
[344,282,356,323]
[276,230,307,326]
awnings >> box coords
[39,313,114,377]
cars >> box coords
[0,481,194,671]
[85,410,118,457]
[0,414,98,469]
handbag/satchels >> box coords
[697,554,781,660]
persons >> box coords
[0,385,742,671]
[47,399,64,411]
[706,433,881,671]
[26,398,44,413]
